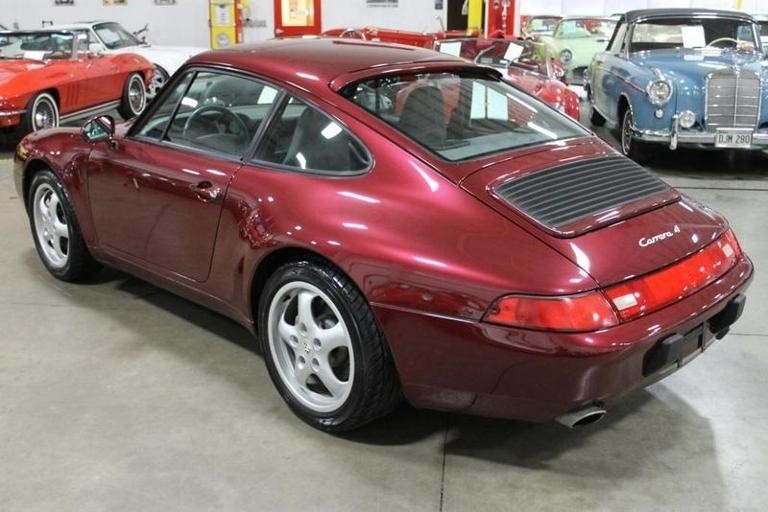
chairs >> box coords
[398,85,448,148]
[282,102,350,170]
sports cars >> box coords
[7,35,761,449]
